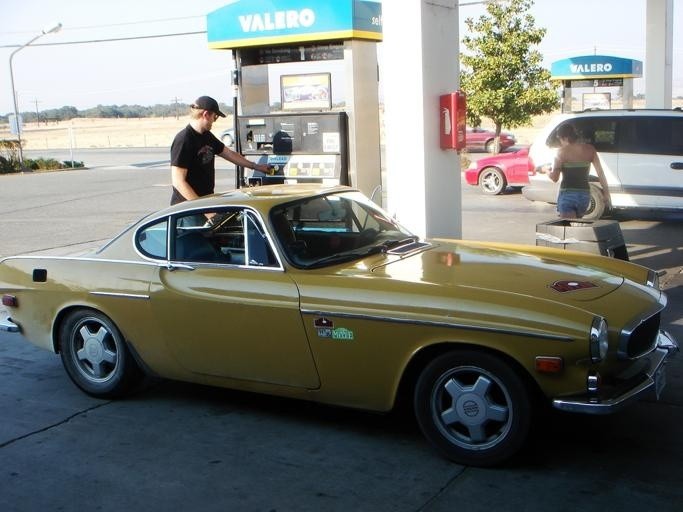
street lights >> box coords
[8,20,63,164]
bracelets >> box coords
[250,161,256,169]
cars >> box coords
[0,182,680,468]
[461,124,517,153]
[216,125,236,147]
[464,142,534,196]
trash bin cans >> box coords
[534,218,629,260]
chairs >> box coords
[175,228,231,264]
[270,215,307,259]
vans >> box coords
[520,107,683,221]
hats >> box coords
[189,96,226,117]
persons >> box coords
[169,93,271,225]
[549,124,612,220]
